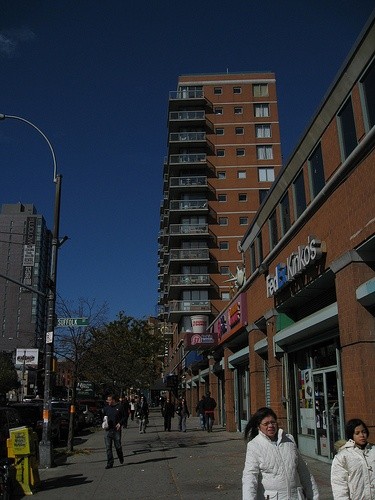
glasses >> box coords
[260,421,277,426]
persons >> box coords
[330,418,375,500]
[196,395,208,430]
[102,394,125,469]
[241,407,320,500]
[134,396,150,434]
[200,392,217,433]
[162,398,175,432]
[118,396,128,429]
[89,393,175,426]
[176,398,190,432]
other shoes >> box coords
[119,455,124,464]
[105,463,113,469]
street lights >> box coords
[0,113,63,441]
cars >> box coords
[27,398,97,419]
